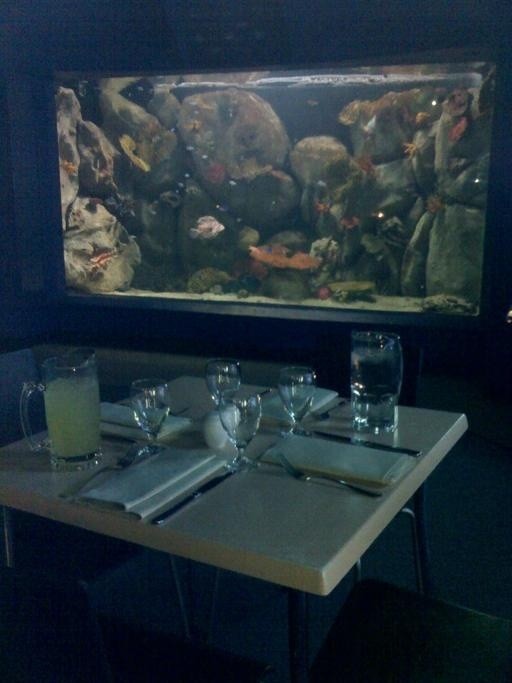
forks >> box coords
[275,450,384,499]
[314,400,346,421]
[59,448,139,499]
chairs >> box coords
[0,572,274,682]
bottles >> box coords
[201,410,229,449]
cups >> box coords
[348,328,405,434]
[18,346,103,472]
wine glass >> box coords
[218,390,263,472]
[274,364,316,440]
[204,357,242,410]
[129,377,170,459]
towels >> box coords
[98,400,193,440]
[260,431,414,486]
[83,440,229,520]
[258,384,339,421]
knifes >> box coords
[151,471,233,525]
[313,428,423,456]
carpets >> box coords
[305,577,512,683]
